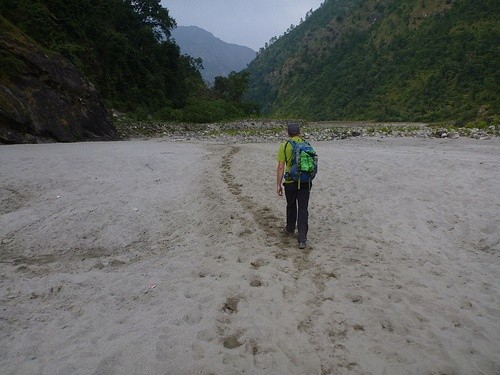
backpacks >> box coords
[283,138,319,185]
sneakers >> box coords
[278,227,294,235]
[298,239,307,249]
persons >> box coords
[276,124,313,249]
